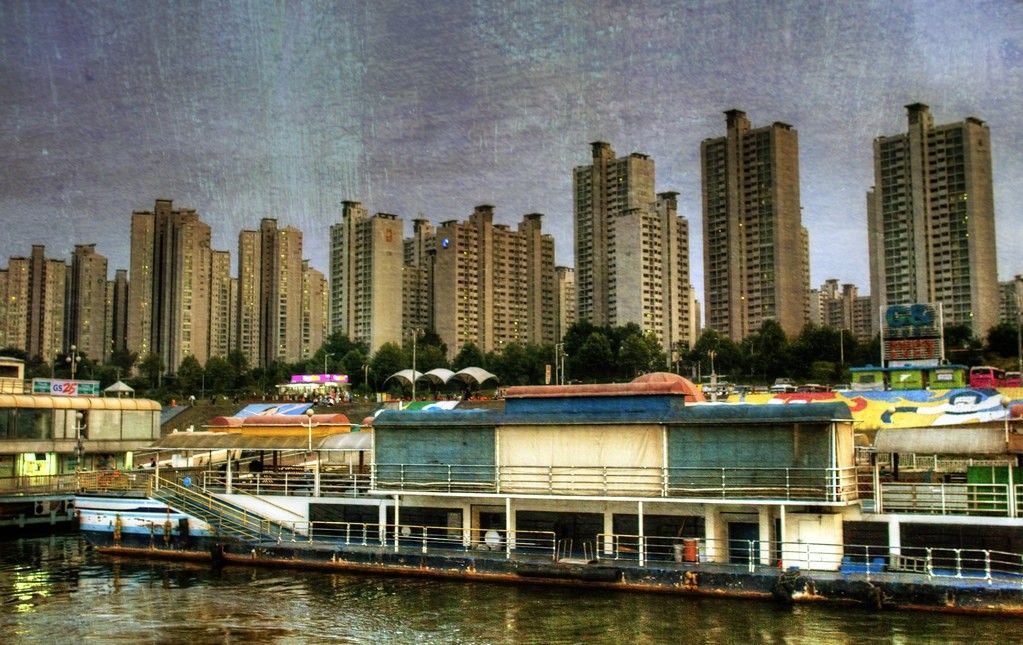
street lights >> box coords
[324,352,335,377]
[841,327,851,367]
[66,344,83,381]
[410,327,427,402]
[362,364,375,393]
[555,342,566,384]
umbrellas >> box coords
[632,373,705,403]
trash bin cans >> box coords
[673,539,699,562]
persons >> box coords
[172,397,177,408]
[189,395,196,404]
[232,394,239,406]
[150,458,157,467]
[284,390,354,407]
[166,463,173,468]
[249,459,262,472]
[305,467,315,496]
[209,394,217,405]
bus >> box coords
[971,366,1007,390]
[1006,370,1021,388]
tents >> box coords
[103,381,135,399]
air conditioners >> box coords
[61,500,74,512]
[34,501,50,515]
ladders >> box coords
[888,553,929,573]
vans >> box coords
[775,378,796,385]
[772,384,797,394]
[798,383,833,395]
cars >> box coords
[831,384,851,392]
